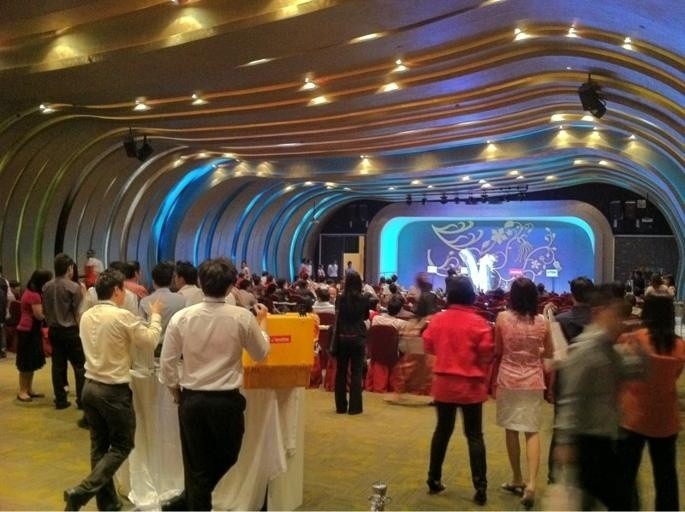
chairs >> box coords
[402,286,579,318]
[6,301,22,353]
[365,325,420,393]
[310,310,353,391]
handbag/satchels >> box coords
[327,326,339,356]
[540,304,571,373]
[537,465,584,512]
[40,320,54,358]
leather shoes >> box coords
[29,391,45,398]
[16,392,32,402]
[101,497,123,511]
[62,486,82,511]
[160,499,174,511]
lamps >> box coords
[125,131,136,158]
[403,189,530,206]
[137,135,153,161]
[576,72,608,120]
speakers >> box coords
[137,143,153,160]
[349,204,368,233]
[609,201,637,235]
[126,140,137,158]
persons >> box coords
[365,267,678,334]
[224,259,360,312]
[0,248,204,429]
[423,277,496,508]
[493,278,548,511]
[546,276,594,485]
[329,271,368,414]
[64,269,163,512]
[616,293,685,512]
[163,257,270,511]
[553,283,646,510]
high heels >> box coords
[472,487,489,505]
[426,478,448,494]
[500,480,526,492]
[520,492,535,506]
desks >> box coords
[397,332,435,394]
[114,363,304,512]
